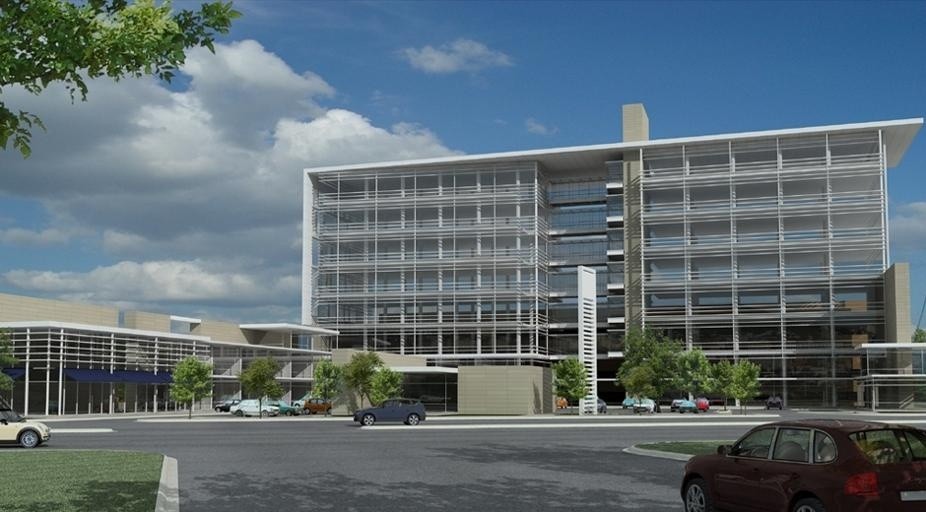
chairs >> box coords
[775,441,804,461]
[869,440,900,464]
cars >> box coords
[304,397,331,414]
[0,396,50,447]
[597,398,606,413]
[672,397,708,413]
[766,394,782,410]
[680,418,926,511]
[217,397,304,417]
[556,396,567,410]
[622,397,656,414]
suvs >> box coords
[352,397,425,425]
